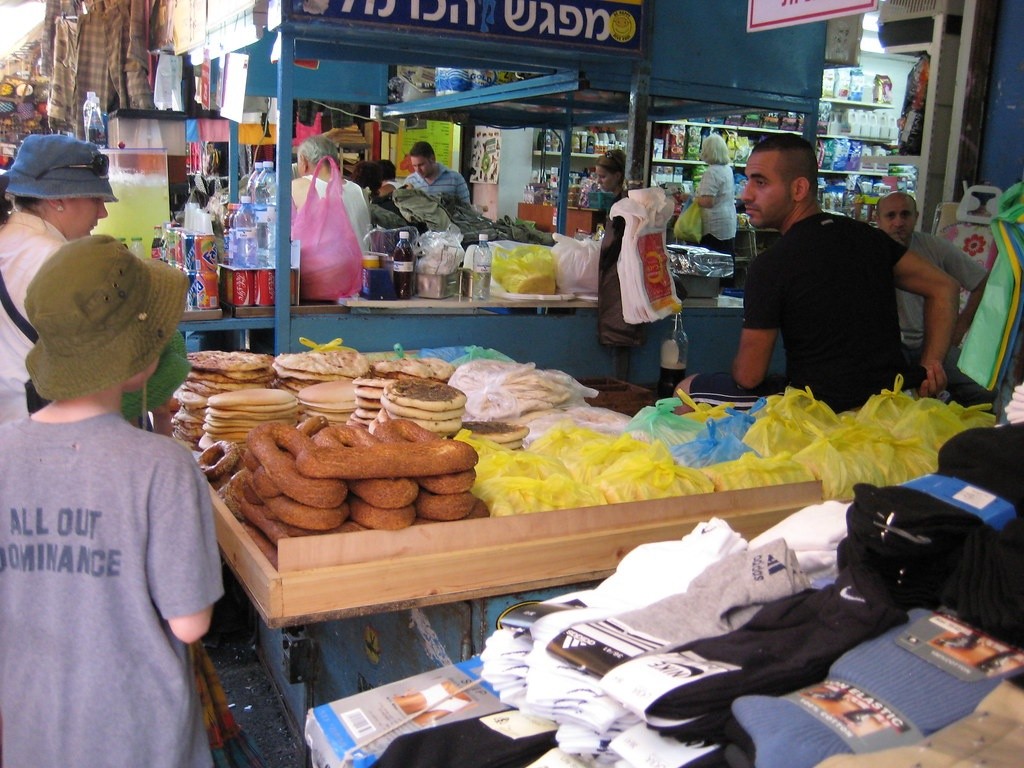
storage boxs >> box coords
[415,273,459,299]
[676,272,719,297]
[108,109,186,156]
[459,268,491,297]
[359,267,398,301]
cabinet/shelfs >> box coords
[517,98,898,290]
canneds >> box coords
[163,229,274,310]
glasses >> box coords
[36,154,110,180]
[604,151,620,165]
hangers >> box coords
[62,12,78,20]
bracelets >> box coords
[692,196,695,203]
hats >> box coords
[24,234,190,401]
[6,134,118,202]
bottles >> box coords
[246,162,263,197]
[472,234,492,301]
[657,313,688,400]
[537,126,628,154]
[393,231,413,300]
[83,91,96,141]
[223,196,275,268]
[88,97,106,148]
[152,225,166,261]
[130,236,145,260]
[254,161,276,196]
[827,107,898,145]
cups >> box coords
[184,203,215,235]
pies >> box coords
[458,430,936,518]
[171,350,639,454]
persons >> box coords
[350,159,407,229]
[596,150,627,270]
[404,141,470,224]
[0,235,225,768]
[875,191,999,408]
[673,133,960,414]
[679,134,737,288]
[291,136,371,254]
[393,680,473,727]
[0,136,119,428]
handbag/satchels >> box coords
[493,232,600,295]
[448,357,995,515]
[292,154,364,299]
[673,197,703,244]
